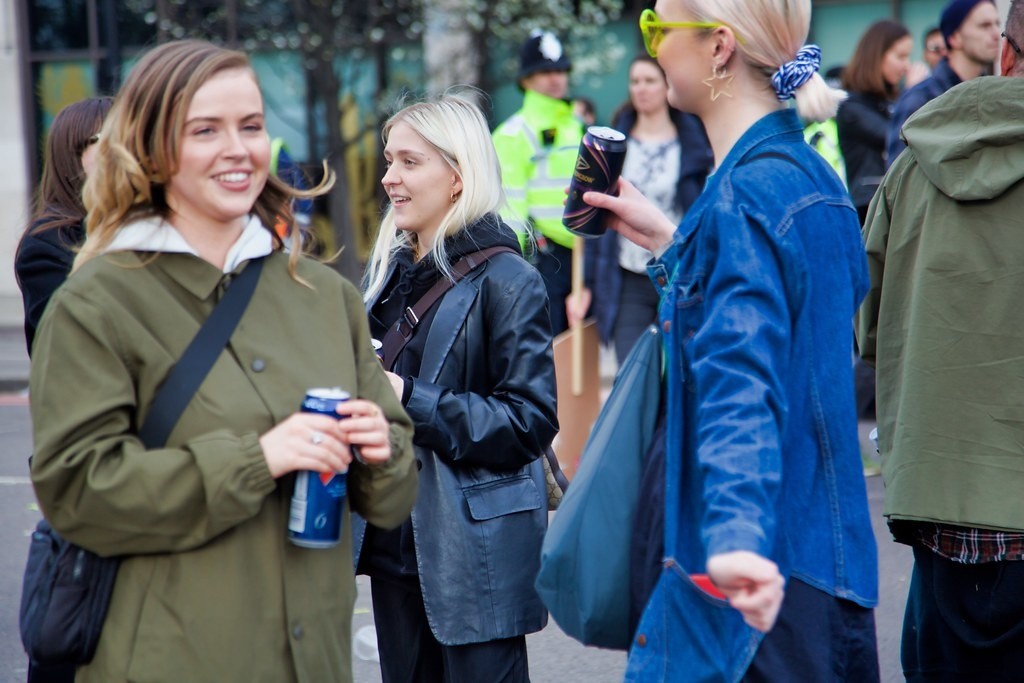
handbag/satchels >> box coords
[541,445,571,509]
[534,316,671,653]
[18,518,119,666]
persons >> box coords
[354,91,559,683]
[15,37,419,683]
[561,1,887,683]
[853,0,1024,683]
[490,0,1003,479]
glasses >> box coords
[639,7,749,58]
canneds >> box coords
[287,387,352,548]
[561,127,628,239]
[371,338,385,369]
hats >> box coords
[513,32,571,81]
[941,0,999,52]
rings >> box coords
[312,431,324,443]
